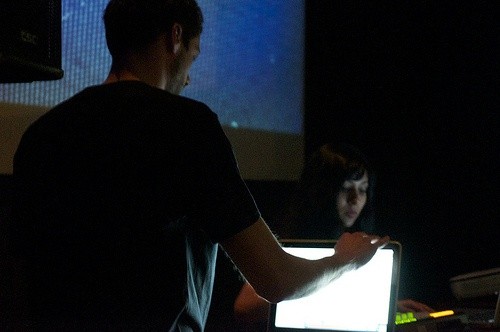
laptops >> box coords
[265,239,401,332]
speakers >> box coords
[0,0,64,84]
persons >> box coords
[233,143,436,332]
[12,0,391,332]
